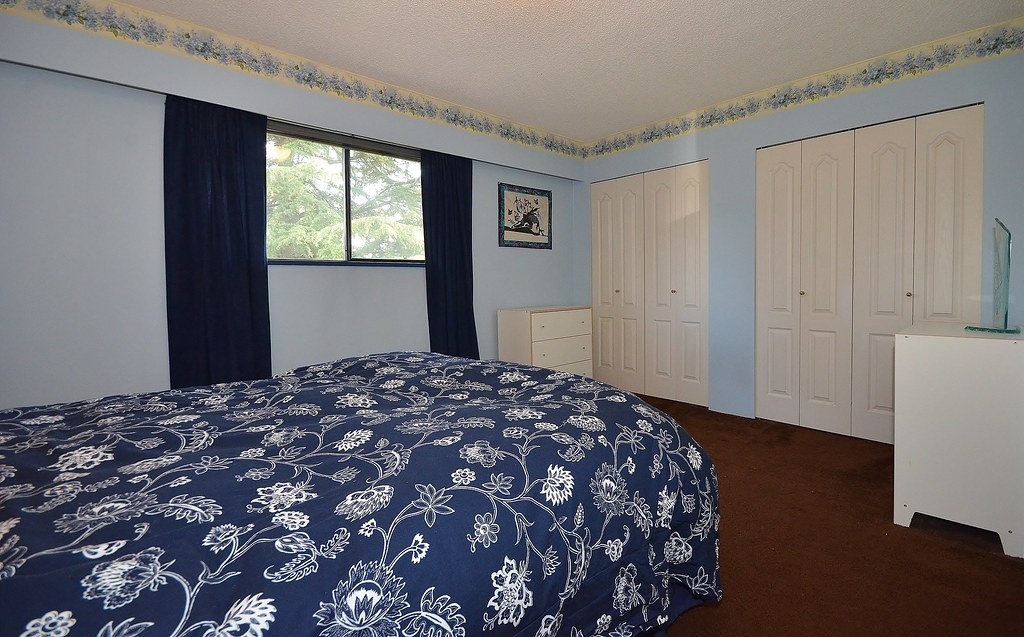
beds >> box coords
[0,349,724,637]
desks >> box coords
[892,321,1024,558]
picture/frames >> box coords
[498,181,553,251]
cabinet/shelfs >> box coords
[497,306,594,380]
[590,159,710,409]
[756,102,985,450]
